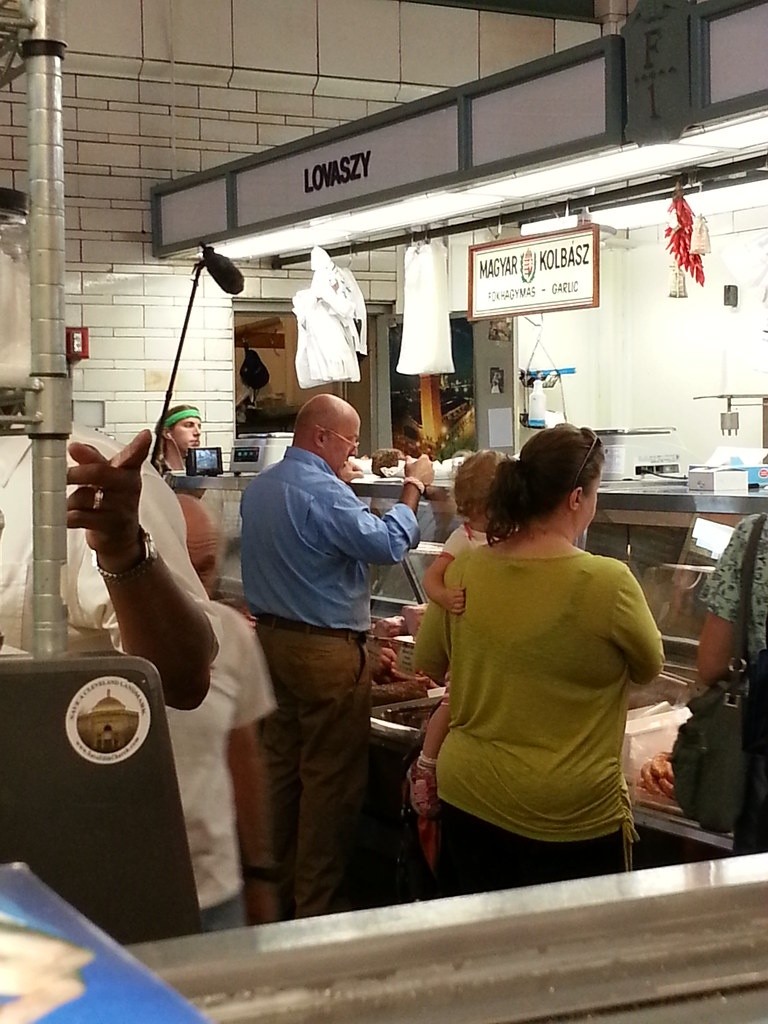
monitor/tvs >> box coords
[187,447,223,474]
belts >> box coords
[258,613,364,639]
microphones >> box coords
[198,241,244,294]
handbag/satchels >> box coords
[666,676,745,833]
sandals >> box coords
[406,762,438,816]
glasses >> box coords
[316,428,360,456]
[555,423,602,498]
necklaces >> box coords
[0,441,33,533]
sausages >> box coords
[641,751,676,801]
[370,647,445,691]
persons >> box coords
[406,448,519,817]
[241,400,434,927]
[0,418,276,944]
[698,512,768,856]
[153,405,202,470]
[414,424,666,900]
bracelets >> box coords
[241,863,274,884]
[96,526,157,585]
[404,475,425,496]
